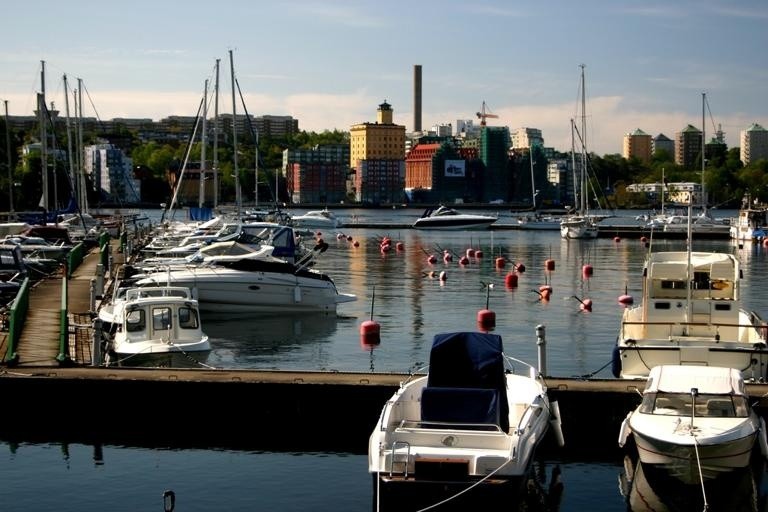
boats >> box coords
[368,373,566,507]
[616,364,768,508]
[412,204,498,230]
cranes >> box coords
[472,100,498,125]
